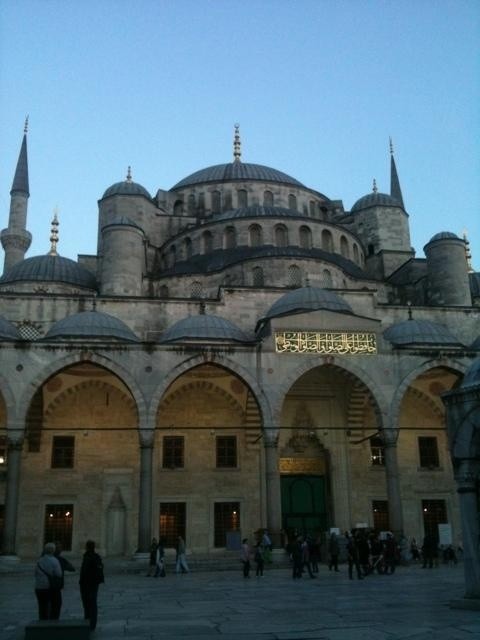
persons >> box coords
[35,543,65,619]
[53,544,77,576]
[238,522,463,583]
[142,533,193,579]
[78,539,105,630]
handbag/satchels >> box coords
[48,575,64,589]
